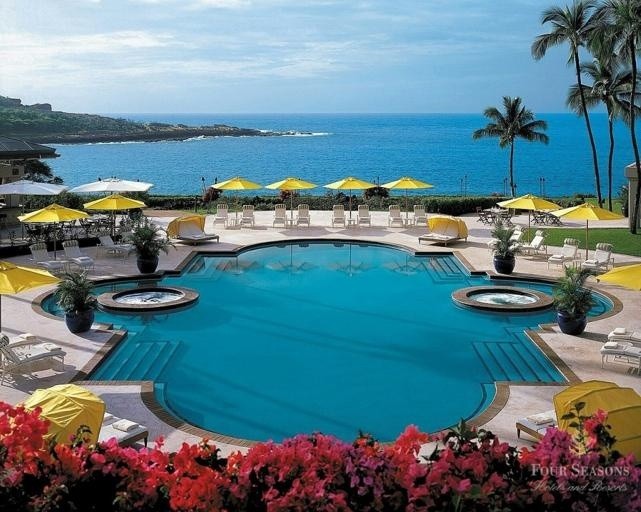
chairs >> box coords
[0,332,67,381]
[582,241,614,273]
[599,342,640,376]
[21,211,115,237]
[474,203,563,226]
[28,242,66,276]
[96,234,131,255]
[62,239,94,272]
[547,238,580,268]
[607,328,640,347]
[210,202,428,228]
[490,224,525,254]
[511,229,546,256]
[8,333,40,349]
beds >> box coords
[6,382,150,459]
[516,379,641,459]
[415,215,468,246]
[168,211,221,246]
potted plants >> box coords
[49,263,98,334]
[552,267,598,338]
[123,179,150,221]
[488,220,521,274]
[124,221,175,274]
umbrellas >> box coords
[595,264,641,292]
[214,256,258,278]
[263,244,320,276]
[327,243,378,279]
[83,192,148,255]
[496,193,564,256]
[1,178,69,242]
[17,203,90,260]
[379,176,436,226]
[66,175,156,235]
[211,176,264,219]
[323,176,378,225]
[265,176,321,228]
[551,201,627,261]
[384,253,428,277]
[1,260,63,334]
